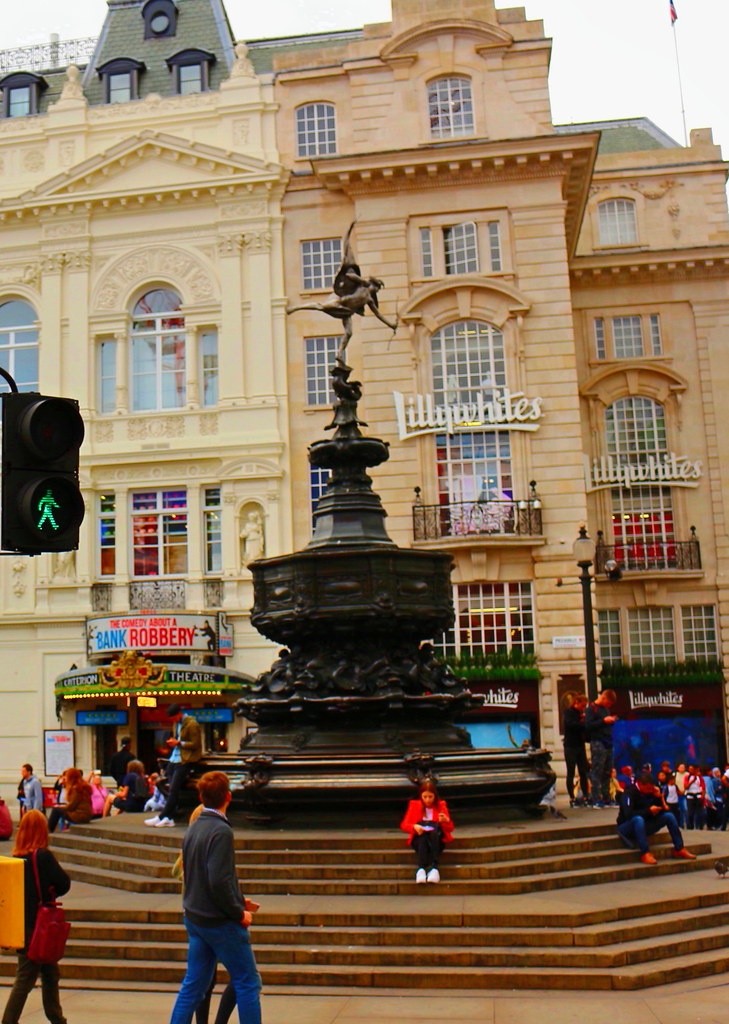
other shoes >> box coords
[641,853,657,864]
[584,800,595,808]
[593,801,606,809]
[673,848,696,859]
[604,802,620,808]
[570,799,581,809]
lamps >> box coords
[604,560,623,582]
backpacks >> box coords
[129,772,148,797]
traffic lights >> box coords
[1,392,85,553]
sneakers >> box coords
[154,817,175,828]
[415,868,426,883]
[426,869,440,883]
[144,816,160,826]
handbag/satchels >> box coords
[27,907,72,965]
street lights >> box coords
[571,518,599,739]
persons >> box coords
[169,770,262,1024]
[401,781,455,883]
[615,771,696,864]
[562,689,729,831]
[0,797,13,841]
[16,736,182,833]
[199,620,216,651]
[144,703,202,827]
[2,809,71,1024]
[240,509,264,562]
[285,273,396,365]
